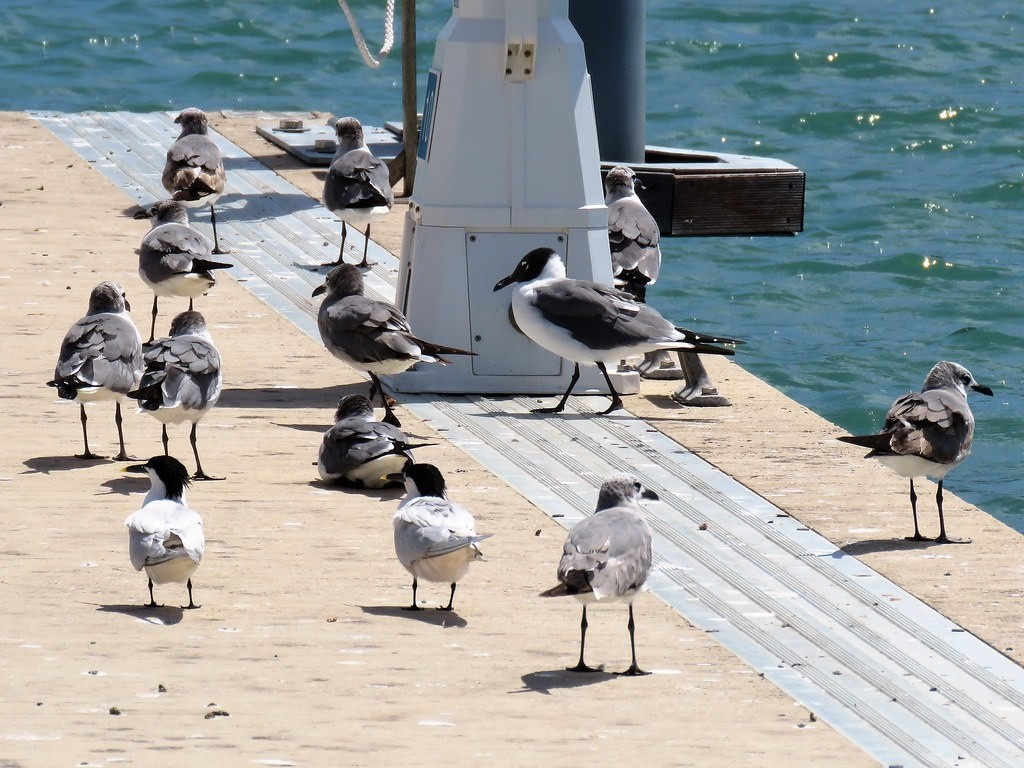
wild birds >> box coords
[491,166,744,416]
[48,201,479,482]
[380,463,492,614]
[162,109,232,256]
[539,474,660,677]
[836,361,994,545]
[323,118,390,265]
[123,456,204,610]
